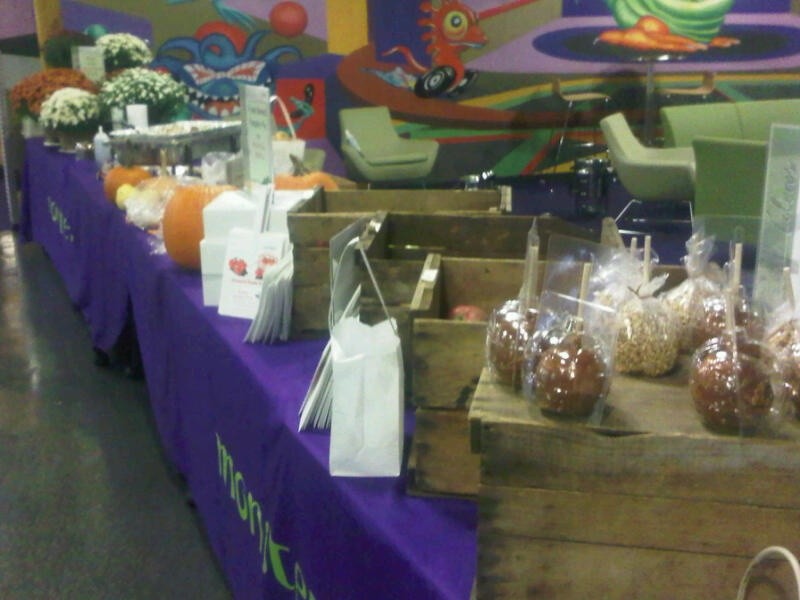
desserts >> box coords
[490,233,800,429]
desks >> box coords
[20,136,479,600]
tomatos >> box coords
[448,305,489,322]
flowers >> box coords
[11,30,186,153]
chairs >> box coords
[601,113,769,247]
[340,106,439,190]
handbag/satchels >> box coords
[328,238,404,477]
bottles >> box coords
[94,125,112,160]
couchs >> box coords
[661,99,800,148]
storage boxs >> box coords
[200,186,800,600]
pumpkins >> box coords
[163,185,240,271]
[105,157,150,203]
[275,154,338,190]
